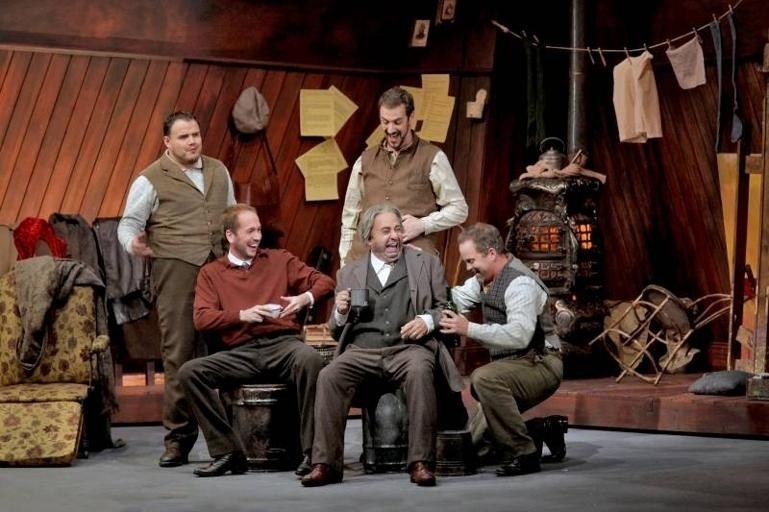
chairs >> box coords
[588,282,731,387]
[0,255,112,468]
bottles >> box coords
[443,286,461,349]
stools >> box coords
[229,383,297,472]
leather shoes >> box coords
[410,461,436,486]
[302,462,344,487]
[295,456,313,476]
[193,449,248,477]
[496,450,541,476]
[540,415,568,462]
[159,448,188,468]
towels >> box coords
[665,36,706,90]
[612,50,663,144]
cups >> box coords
[348,288,370,309]
[262,303,283,322]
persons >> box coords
[336,85,470,270]
[300,202,451,486]
[116,109,238,468]
[438,220,570,477]
[177,202,336,477]
